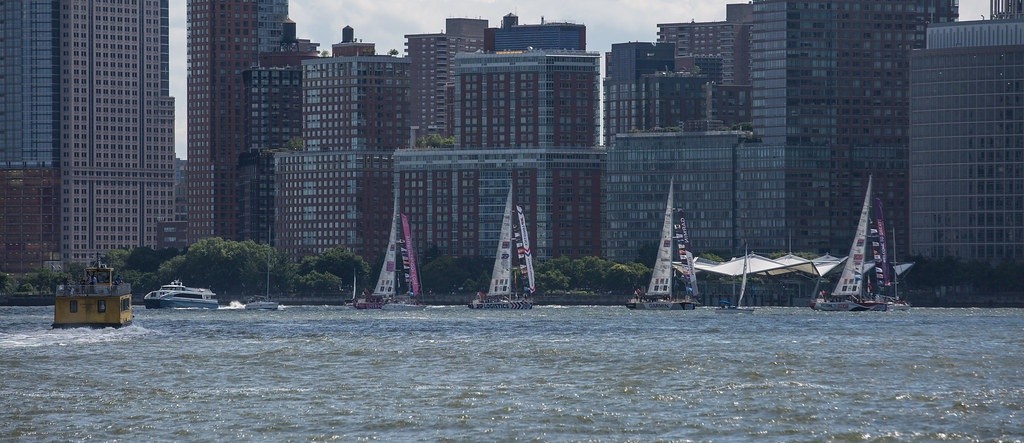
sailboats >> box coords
[809,174,887,311]
[342,187,427,310]
[468,179,534,310]
[714,239,755,314]
[626,173,697,310]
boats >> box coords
[245,295,280,310]
[52,251,133,328]
[143,280,219,310]
